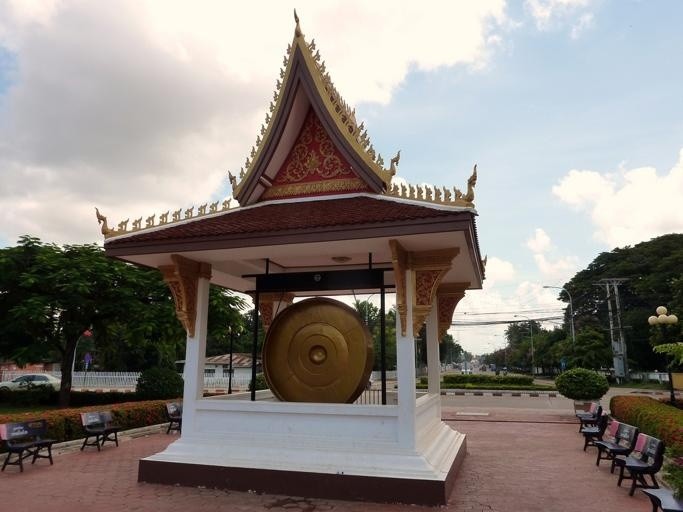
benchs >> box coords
[575,402,683,512]
[0,409,122,472]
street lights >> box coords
[648,306,678,405]
[543,285,577,368]
[224,325,244,394]
[514,314,536,381]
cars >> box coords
[0,373,62,391]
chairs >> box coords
[163,402,182,434]
